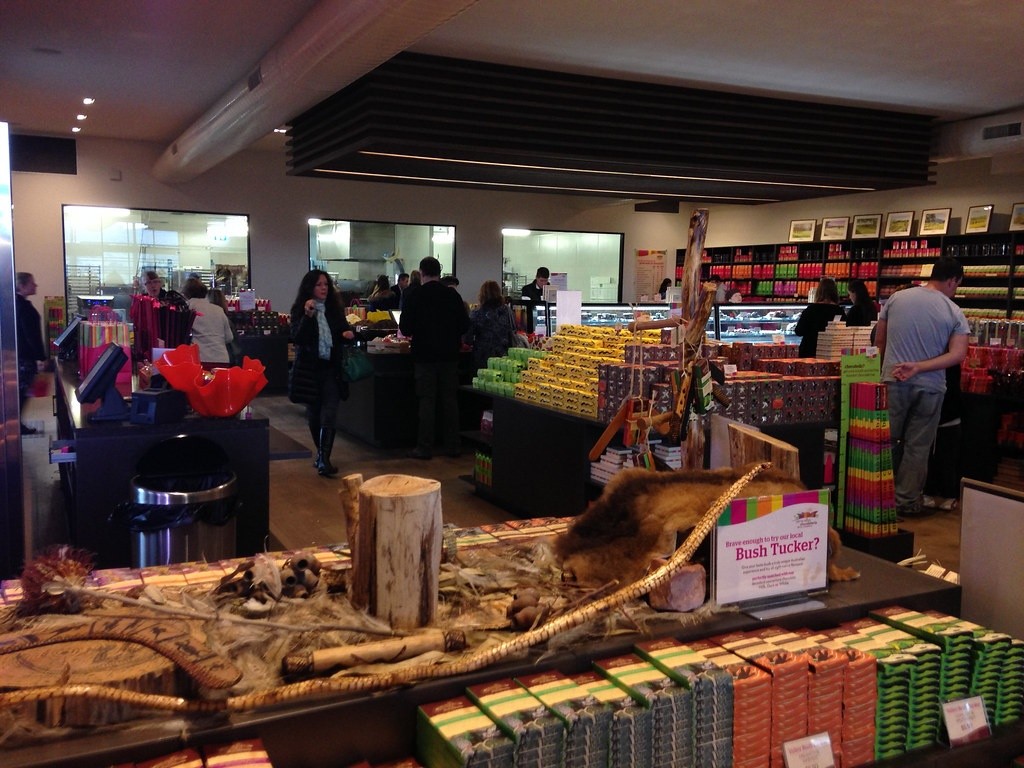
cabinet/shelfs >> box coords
[675,229,1024,322]
[227,335,288,394]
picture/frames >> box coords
[1008,202,1024,232]
[820,216,850,240]
[919,208,952,237]
[851,214,883,239]
[788,219,817,242]
[884,211,915,237]
[965,204,994,234]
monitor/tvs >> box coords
[543,285,560,302]
[74,342,129,404]
[52,313,88,348]
[389,309,402,326]
[666,287,682,303]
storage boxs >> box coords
[473,324,842,430]
[417,605,1024,768]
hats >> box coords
[144,271,162,285]
[189,273,202,279]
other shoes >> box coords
[922,494,937,508]
[897,503,938,518]
[939,498,959,511]
[406,447,432,460]
[20,421,36,435]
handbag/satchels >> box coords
[23,370,56,398]
[511,335,531,349]
[340,341,375,383]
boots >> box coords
[318,423,339,476]
[307,423,320,468]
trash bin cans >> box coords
[128,434,238,568]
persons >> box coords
[710,275,743,303]
[287,268,357,476]
[399,257,471,459]
[659,278,672,300]
[463,280,518,384]
[870,256,971,516]
[846,280,877,326]
[144,271,237,372]
[367,270,461,312]
[521,267,550,301]
[794,279,850,358]
[15,272,46,435]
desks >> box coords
[0,544,960,768]
[457,385,841,520]
[336,348,482,448]
